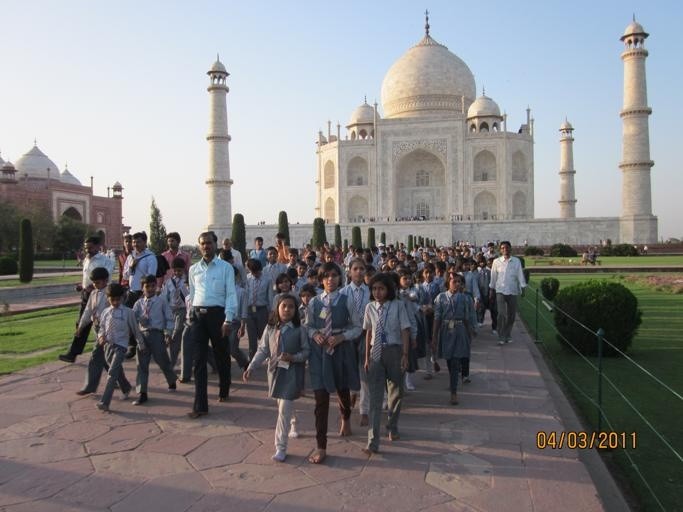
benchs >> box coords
[582,260,602,266]
[534,260,554,266]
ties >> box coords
[426,284,433,305]
[269,327,283,375]
[106,311,118,345]
[92,292,100,326]
[144,299,153,331]
[372,307,385,362]
[452,295,457,312]
[325,296,335,356]
[356,287,364,323]
[174,277,181,309]
[252,278,258,306]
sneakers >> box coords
[187,411,207,417]
[271,450,285,462]
[168,382,176,391]
[434,362,440,372]
[217,398,227,402]
[492,329,512,344]
[76,388,147,410]
[382,402,389,410]
[407,381,414,390]
[59,353,76,363]
[424,373,432,379]
[462,377,471,383]
[289,418,300,437]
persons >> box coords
[633,244,637,248]
[581,245,602,265]
[59,233,528,465]
[256,213,496,226]
[642,244,648,256]
[599,238,611,248]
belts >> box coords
[197,307,224,314]
